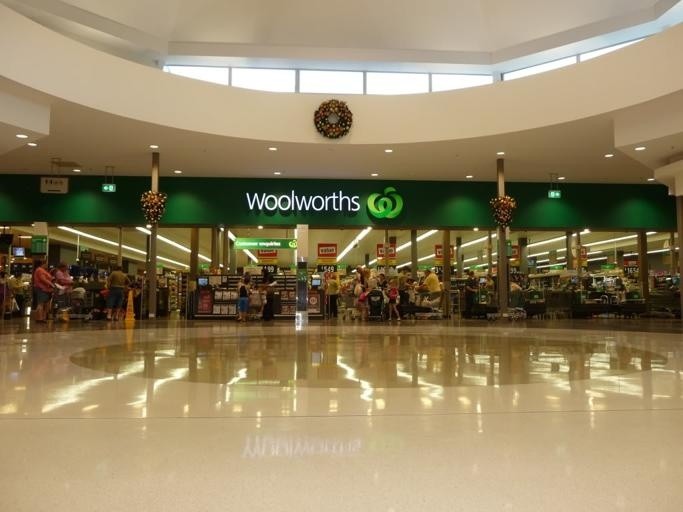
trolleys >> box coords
[47,282,89,323]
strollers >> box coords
[338,286,363,321]
[364,288,388,321]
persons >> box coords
[13,272,26,314]
[322,256,445,322]
[464,271,477,318]
[33,261,53,323]
[485,274,493,289]
[104,264,131,320]
[260,267,275,321]
[56,261,71,306]
[616,271,626,297]
[53,277,67,294]
[236,273,251,322]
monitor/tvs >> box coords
[197,277,209,287]
[311,280,321,286]
[12,246,25,257]
